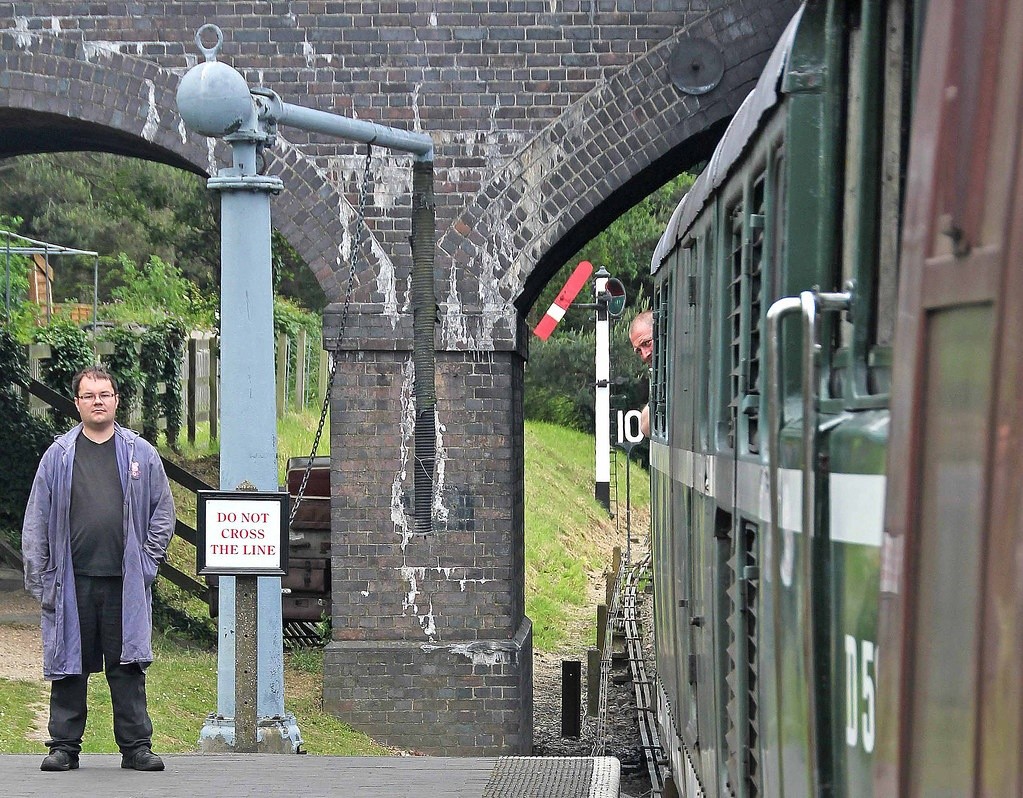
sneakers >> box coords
[121,745,164,771]
[41,749,79,771]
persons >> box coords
[20,366,176,773]
[628,308,655,440]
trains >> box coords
[610,0,1023,798]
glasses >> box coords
[78,390,115,402]
